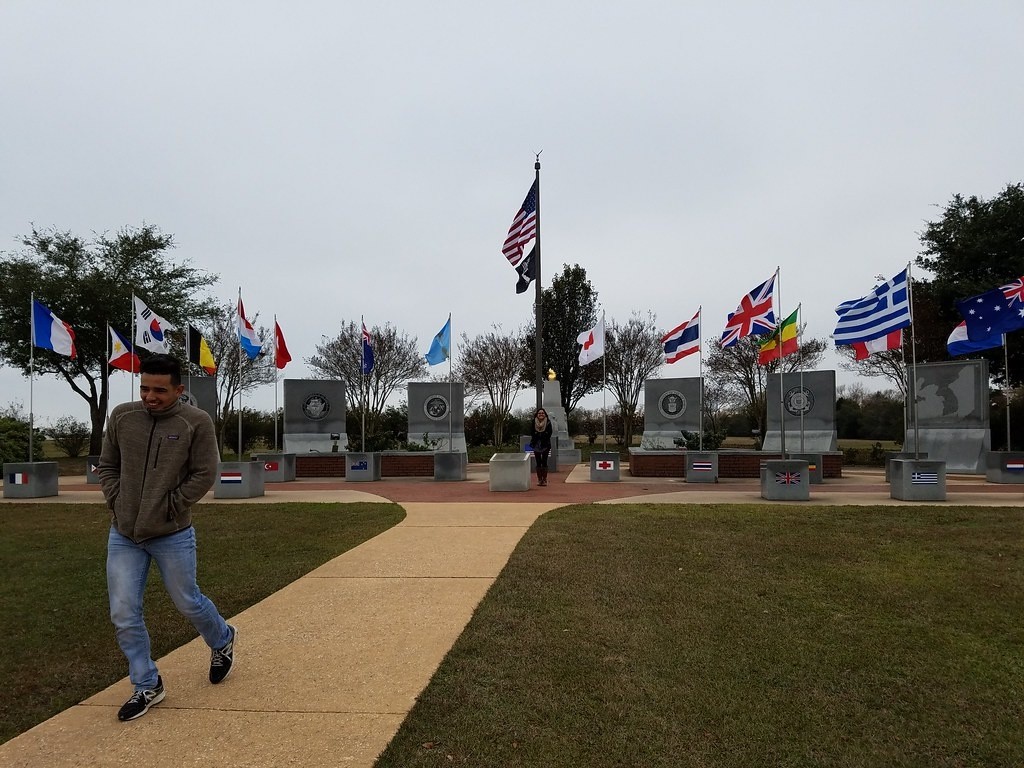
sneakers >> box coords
[117,674,165,721]
[208,624,236,684]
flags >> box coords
[832,267,912,362]
[756,306,800,366]
[577,316,604,366]
[947,276,1024,358]
[273,320,292,370]
[108,325,142,373]
[660,309,701,364]
[501,178,537,294]
[425,318,451,365]
[188,323,216,375]
[721,269,778,351]
[237,297,262,362]
[32,298,76,360]
[134,295,173,354]
[359,322,374,374]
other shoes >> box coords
[542,480,547,486]
[537,479,542,486]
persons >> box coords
[97,354,239,721]
[529,408,553,486]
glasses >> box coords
[538,413,544,414]
